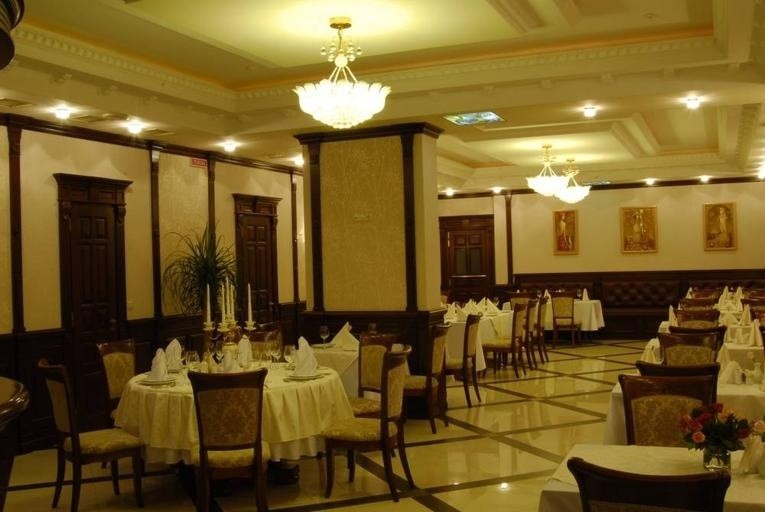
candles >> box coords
[224,276,230,317]
[229,286,235,321]
[206,282,212,328]
[247,283,253,322]
[221,283,225,328]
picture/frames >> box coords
[618,205,660,254]
[702,202,738,251]
[553,210,579,256]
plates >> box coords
[311,343,333,348]
[453,318,467,323]
[284,362,320,370]
[137,380,173,385]
[485,312,498,316]
[284,375,324,380]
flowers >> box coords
[673,403,753,470]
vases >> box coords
[702,449,733,469]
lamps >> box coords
[292,17,393,130]
[524,143,570,196]
[555,156,592,205]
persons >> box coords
[719,208,727,234]
[556,212,571,250]
[631,209,645,244]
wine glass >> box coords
[257,343,282,370]
[283,345,296,377]
[202,351,212,369]
[185,351,200,369]
[577,288,582,301]
[491,297,499,308]
[452,302,461,317]
[536,290,542,299]
[319,325,329,352]
[368,322,377,333]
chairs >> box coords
[550,292,582,348]
[443,312,483,408]
[0,381,35,512]
[533,295,549,364]
[321,344,416,503]
[404,321,451,434]
[740,299,764,308]
[504,299,537,370]
[39,358,146,511]
[666,324,727,343]
[185,370,271,511]
[634,358,719,376]
[94,338,139,470]
[617,374,719,448]
[481,302,527,378]
[679,298,714,309]
[688,287,720,298]
[657,332,718,365]
[344,338,398,459]
[675,310,721,328]
[742,287,764,297]
[566,457,731,512]
[748,307,764,319]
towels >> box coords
[737,287,743,298]
[469,302,478,315]
[446,304,454,320]
[486,298,500,314]
[165,339,182,372]
[728,303,736,311]
[720,344,730,375]
[729,313,738,324]
[735,328,743,344]
[740,436,762,474]
[477,298,485,312]
[294,337,317,376]
[464,300,478,315]
[456,304,466,321]
[739,305,750,326]
[749,320,763,346]
[641,348,656,364]
[723,286,728,300]
[457,307,466,323]
[151,348,167,381]
[718,311,722,324]
[582,288,590,302]
[544,290,551,302]
[332,323,351,344]
[238,335,252,368]
[669,305,678,327]
[724,327,732,343]
[721,361,740,383]
[342,330,360,351]
[686,292,692,298]
[447,302,455,317]
[719,295,725,309]
[758,443,764,477]
[689,287,692,290]
